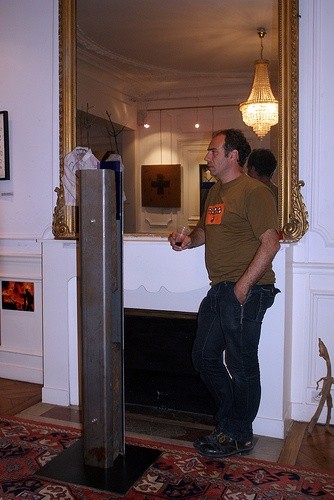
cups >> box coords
[174,225,191,246]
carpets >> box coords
[0,413,334,500]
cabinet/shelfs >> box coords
[141,107,280,238]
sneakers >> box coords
[194,428,254,457]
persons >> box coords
[169,129,281,456]
[248,149,278,204]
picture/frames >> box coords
[1,112,11,180]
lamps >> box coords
[240,33,278,142]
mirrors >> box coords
[50,0,308,243]
